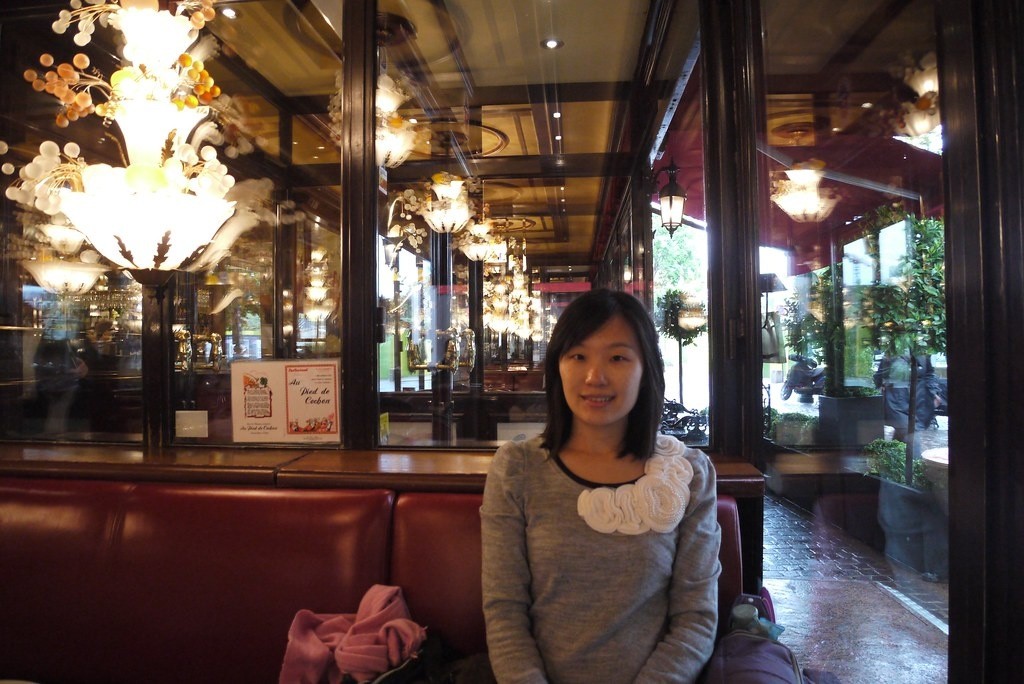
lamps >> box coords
[645,161,693,236]
[426,55,545,343]
[329,13,418,168]
[891,53,949,154]
[0,0,255,288]
[770,131,843,221]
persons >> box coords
[477,286,727,684]
[872,338,940,461]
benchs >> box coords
[0,481,802,683]
